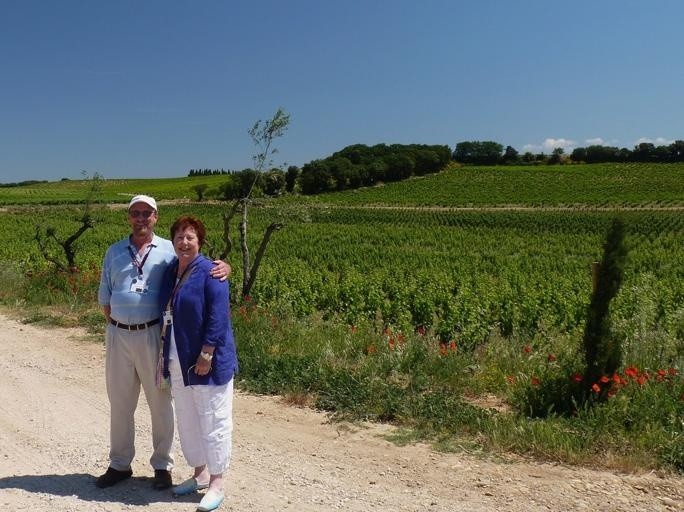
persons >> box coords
[155,215,241,512]
[94,194,235,490]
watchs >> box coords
[200,351,214,362]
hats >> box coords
[127,194,158,212]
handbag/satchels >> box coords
[155,356,170,391]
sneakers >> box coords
[172,477,209,496]
[96,466,133,488]
[153,468,171,489]
[198,489,226,511]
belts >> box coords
[110,317,162,330]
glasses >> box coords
[129,210,156,218]
